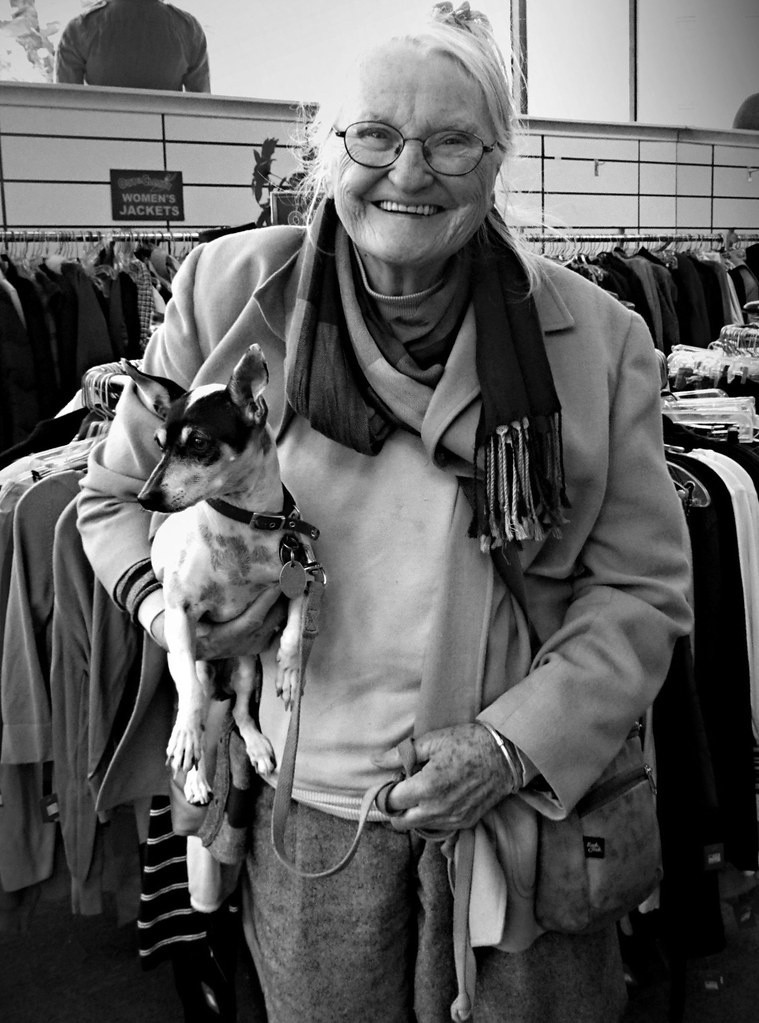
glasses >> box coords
[332,121,498,175]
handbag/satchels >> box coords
[539,722,664,936]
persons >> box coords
[53,0,210,94]
[75,2,695,1023]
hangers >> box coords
[0,225,759,260]
[655,321,759,508]
[75,360,142,438]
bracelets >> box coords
[480,721,521,795]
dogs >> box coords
[120,342,316,807]
